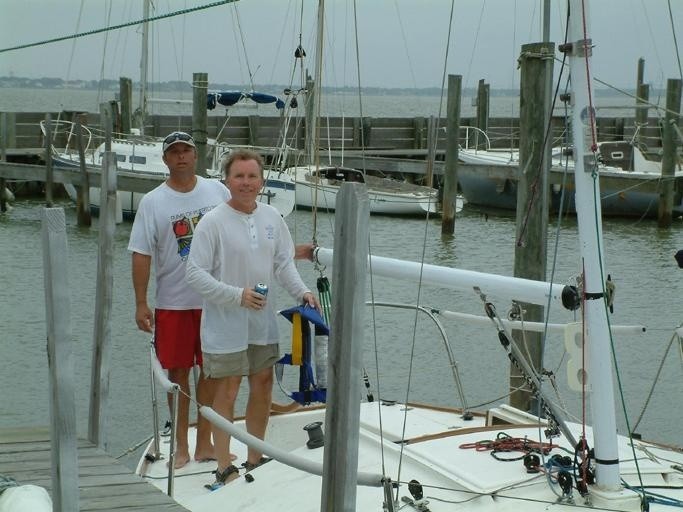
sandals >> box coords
[241,457,272,472]
[212,465,238,484]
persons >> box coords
[122,128,314,474]
[186,141,322,491]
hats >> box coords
[162,131,196,155]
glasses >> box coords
[162,134,195,148]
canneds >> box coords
[255,281,268,306]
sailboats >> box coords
[136,0,682,512]
[0,0,681,222]
